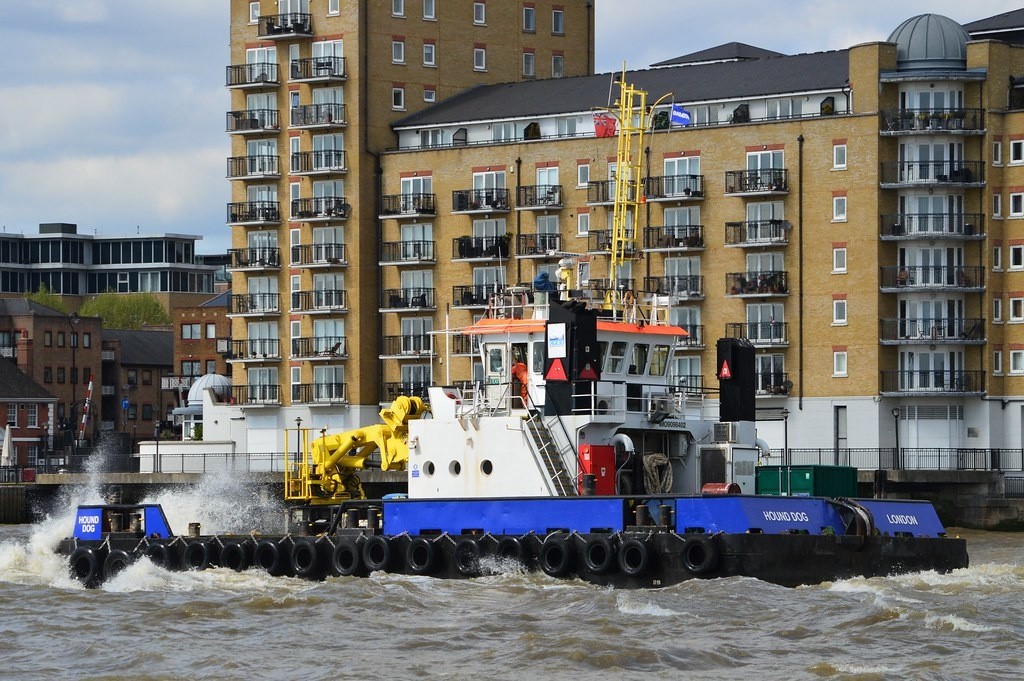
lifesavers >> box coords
[287,538,319,579]
[66,545,98,586]
[616,539,650,577]
[582,535,616,573]
[452,537,484,576]
[182,540,212,574]
[679,536,722,575]
[100,549,135,585]
[539,538,575,577]
[141,543,173,573]
[361,536,393,571]
[493,535,527,572]
[405,537,436,574]
[252,539,284,576]
[331,540,360,577]
[219,541,249,572]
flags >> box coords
[671,104,692,126]
[594,111,616,138]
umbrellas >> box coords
[1,425,14,483]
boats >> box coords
[48,63,970,588]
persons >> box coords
[511,354,528,409]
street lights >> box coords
[67,310,81,438]
[779,409,792,466]
[891,408,902,470]
[41,421,50,474]
[294,417,303,472]
[154,420,161,474]
